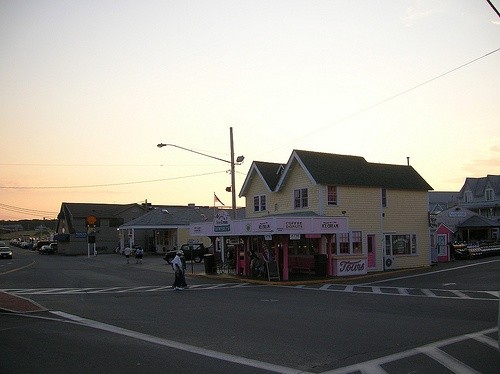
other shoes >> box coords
[173,286,184,291]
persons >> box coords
[135,247,144,264]
[171,250,189,290]
[125,245,132,264]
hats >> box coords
[176,251,181,254]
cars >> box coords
[0,236,58,260]
[162,241,206,265]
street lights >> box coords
[155,126,245,219]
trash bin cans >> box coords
[203,253,218,275]
[314,254,328,276]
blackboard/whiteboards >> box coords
[266,259,281,282]
